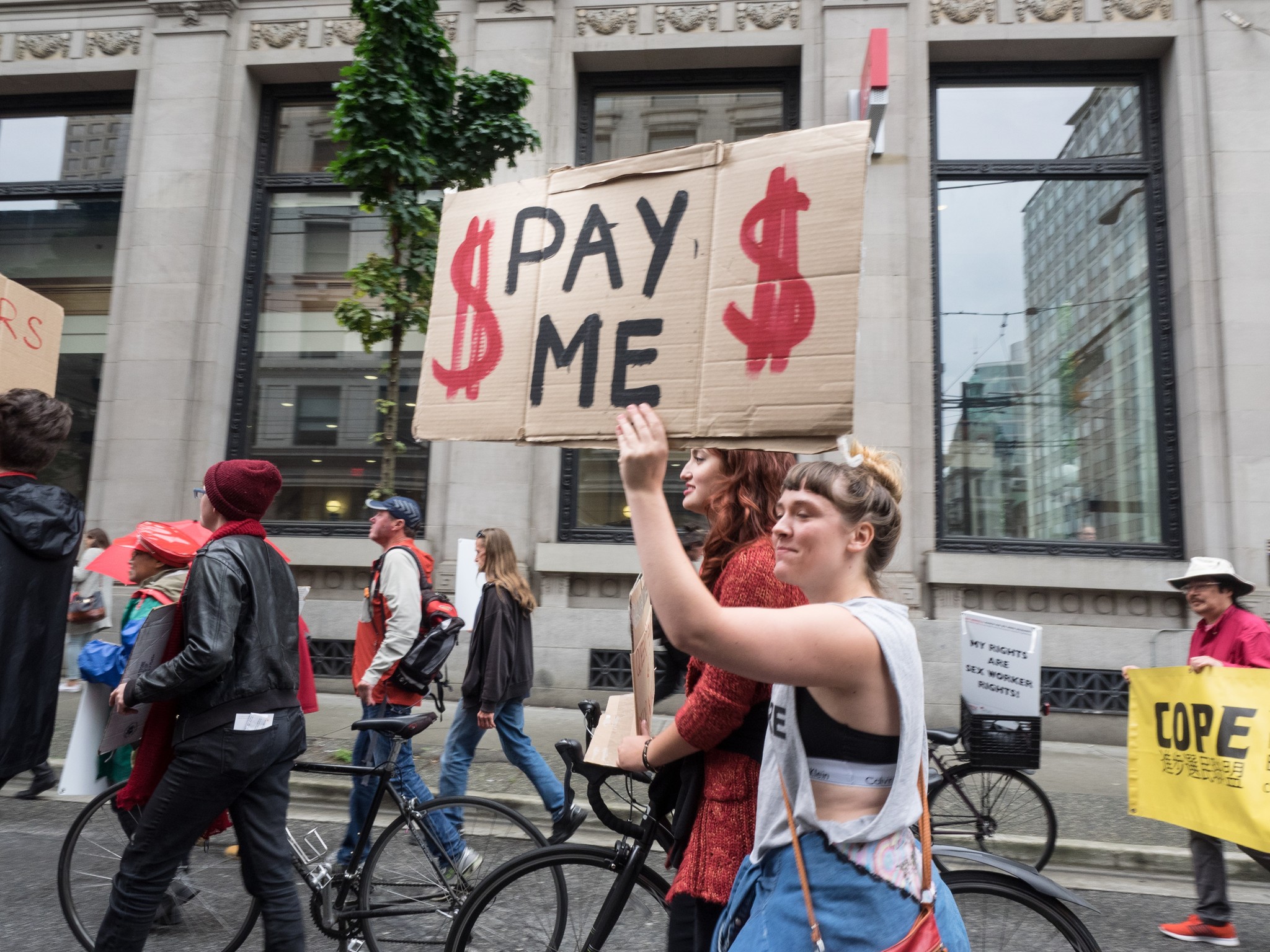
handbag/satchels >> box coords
[66,591,105,623]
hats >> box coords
[363,496,420,531]
[133,520,201,568]
[204,459,283,521]
[1165,556,1256,597]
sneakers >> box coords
[1157,914,1240,948]
[172,871,201,902]
[15,762,59,801]
[546,805,587,843]
[151,894,182,932]
[432,853,484,899]
[409,817,465,846]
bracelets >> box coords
[640,736,656,774]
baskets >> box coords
[958,696,1041,768]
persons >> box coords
[58,527,114,691]
[92,460,309,952]
[615,400,973,952]
[75,519,204,928]
[617,447,809,952]
[0,388,87,788]
[405,526,588,852]
[1120,555,1270,951]
[306,498,485,904]
[16,762,57,800]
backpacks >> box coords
[385,588,465,695]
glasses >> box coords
[193,488,208,500]
[1179,582,1224,594]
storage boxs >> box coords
[959,694,1041,770]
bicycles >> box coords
[445,699,1102,952]
[924,695,1058,874]
[56,711,568,952]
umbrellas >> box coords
[85,518,293,591]
